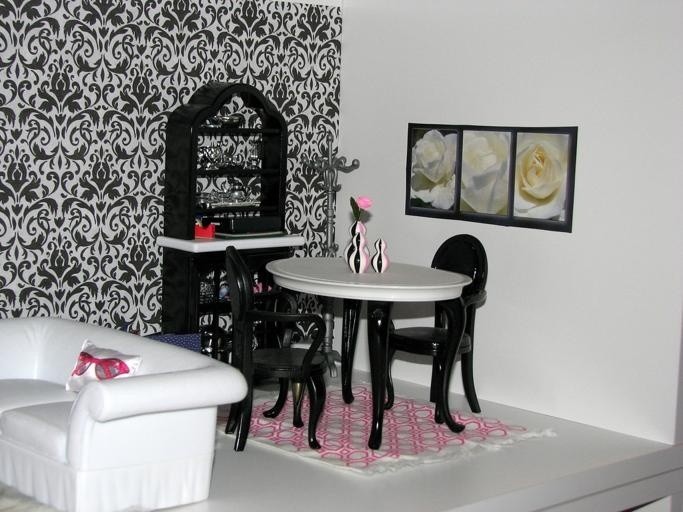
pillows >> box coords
[65,334,140,400]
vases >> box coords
[347,231,370,274]
[342,220,368,263]
[370,237,389,273]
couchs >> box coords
[0,312,249,511]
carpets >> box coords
[214,378,561,477]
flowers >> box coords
[346,194,372,224]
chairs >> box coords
[381,231,488,436]
[209,245,332,457]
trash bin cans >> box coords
[197,325,232,365]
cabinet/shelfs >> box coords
[157,78,304,339]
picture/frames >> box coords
[402,121,579,233]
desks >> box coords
[255,255,474,453]
